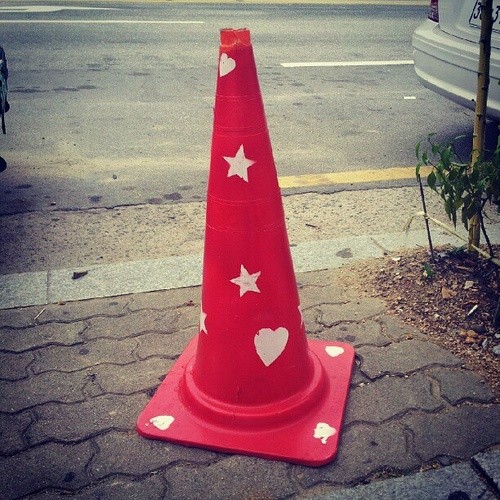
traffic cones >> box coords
[137,24,357,466]
